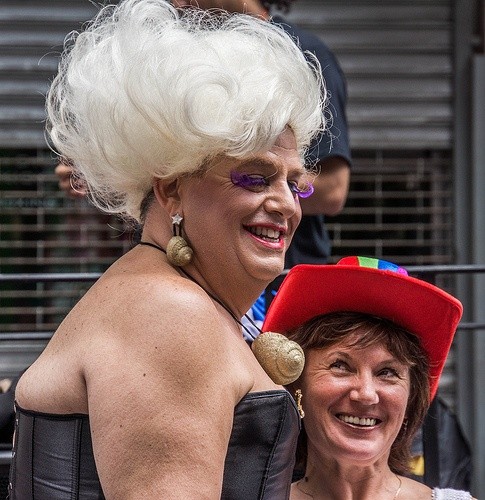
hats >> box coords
[261,256,463,406]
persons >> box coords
[6,1,336,500]
[261,255,479,500]
[55,0,351,348]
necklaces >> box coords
[134,238,305,385]
